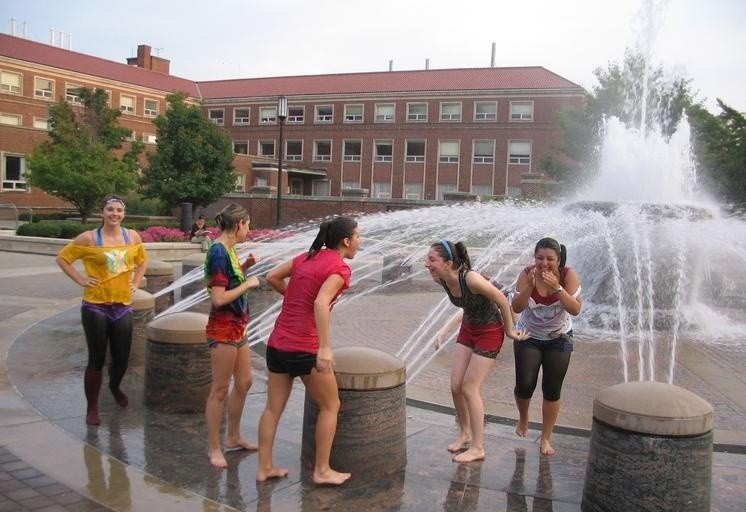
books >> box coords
[194,229,210,237]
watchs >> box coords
[553,285,562,294]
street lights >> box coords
[273,92,288,230]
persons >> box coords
[201,202,262,471]
[424,238,533,465]
[510,237,585,459]
[254,217,361,486]
[56,195,149,426]
[189,215,212,253]
